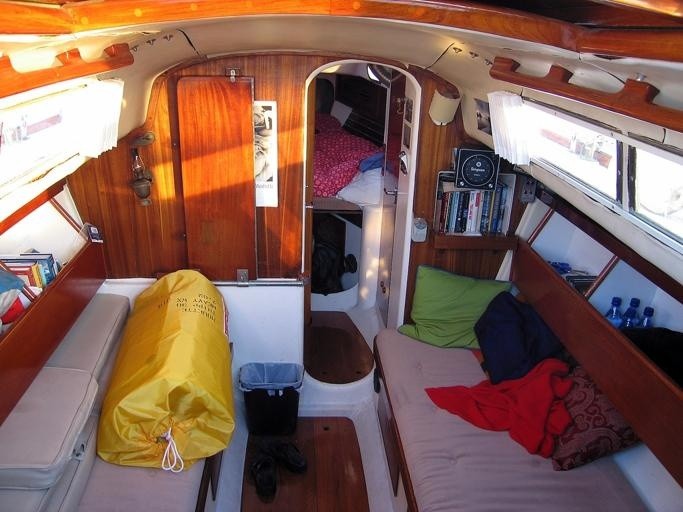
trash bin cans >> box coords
[239,362,304,434]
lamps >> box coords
[127,146,153,211]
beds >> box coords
[373,328,652,512]
[313,77,384,211]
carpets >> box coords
[305,310,373,385]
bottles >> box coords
[602,296,656,331]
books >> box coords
[0,248,62,290]
[433,174,508,237]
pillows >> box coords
[396,261,513,351]
[330,100,354,128]
[550,363,642,471]
